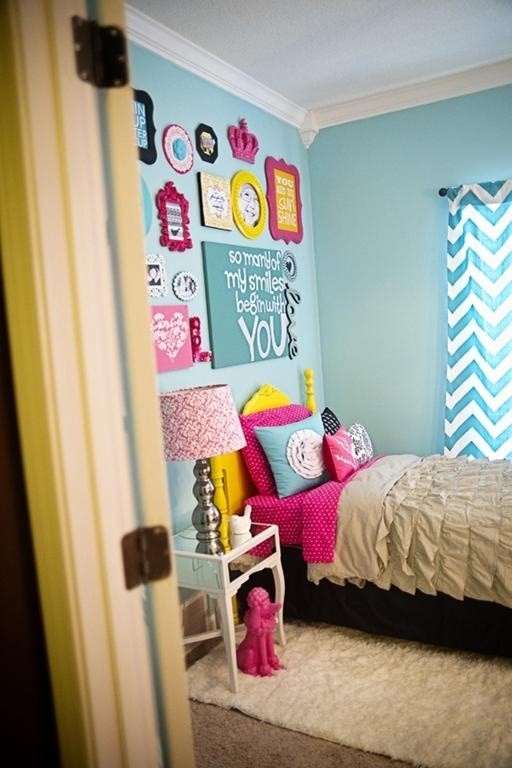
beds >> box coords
[216,369,512,622]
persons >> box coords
[148,264,161,285]
[239,183,260,227]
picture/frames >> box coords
[199,170,232,231]
[155,181,193,252]
[172,271,199,302]
[231,170,267,239]
[264,156,304,245]
[146,254,168,298]
[195,123,218,164]
[132,88,157,165]
[162,124,194,175]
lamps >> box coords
[158,383,247,540]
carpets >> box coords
[185,617,512,767]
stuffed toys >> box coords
[236,587,281,677]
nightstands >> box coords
[173,519,287,691]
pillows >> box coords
[239,404,375,499]
